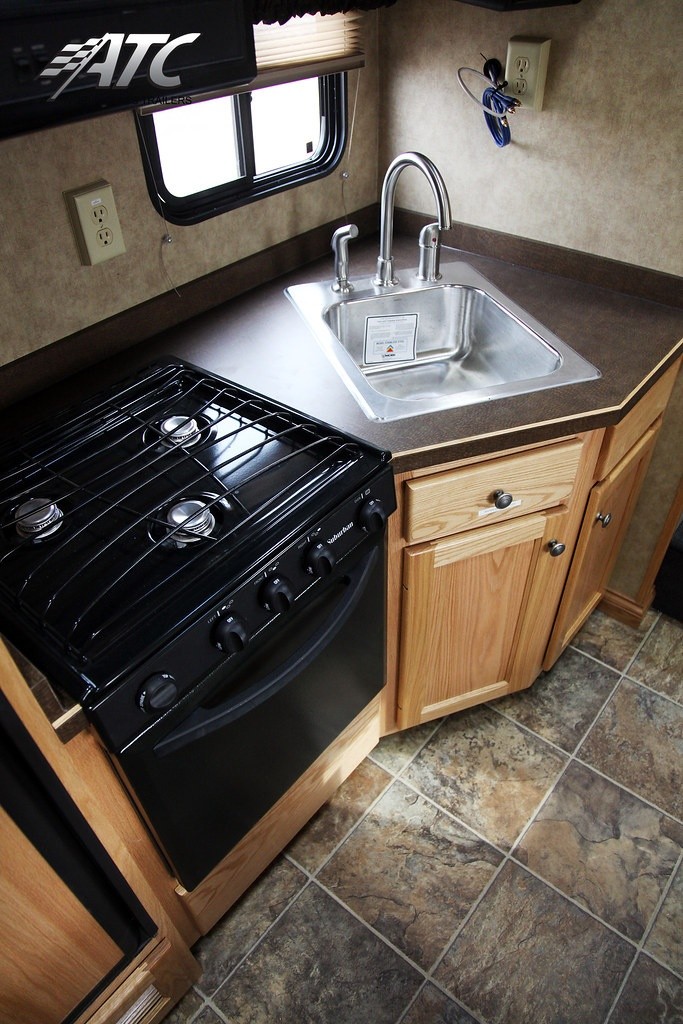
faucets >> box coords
[371,151,452,286]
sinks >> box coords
[283,261,603,424]
